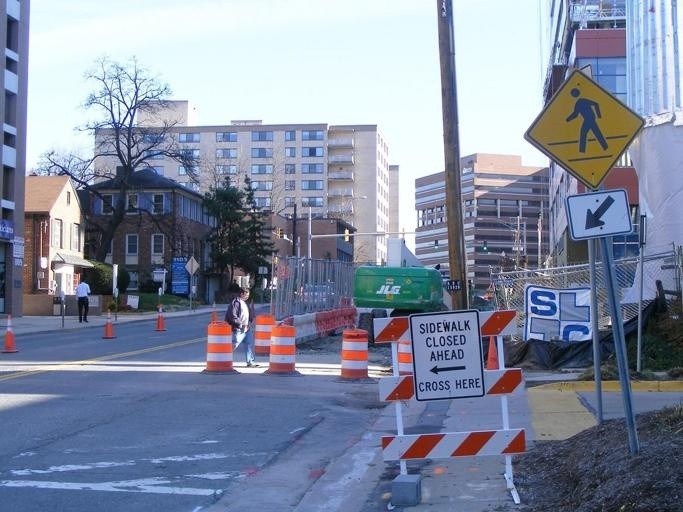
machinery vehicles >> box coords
[350,263,452,348]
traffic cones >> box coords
[209,300,219,323]
[1,313,20,354]
[102,309,118,340]
[155,304,168,332]
[486,335,500,370]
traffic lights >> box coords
[435,240,438,249]
[484,241,487,251]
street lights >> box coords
[306,194,369,284]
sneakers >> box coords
[247,363,261,368]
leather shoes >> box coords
[84,320,88,323]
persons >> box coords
[75,276,91,324]
[225,288,260,368]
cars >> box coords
[300,282,336,311]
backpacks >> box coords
[224,304,232,324]
